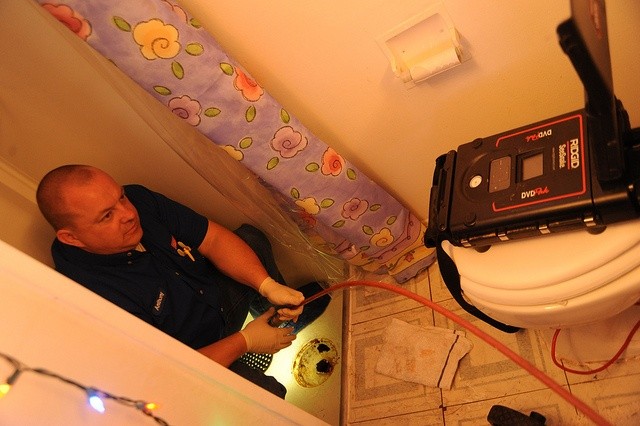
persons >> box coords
[35,164,333,404]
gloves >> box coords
[260,277,305,323]
[238,307,296,354]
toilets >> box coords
[451,221,640,365]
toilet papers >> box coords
[409,46,463,86]
